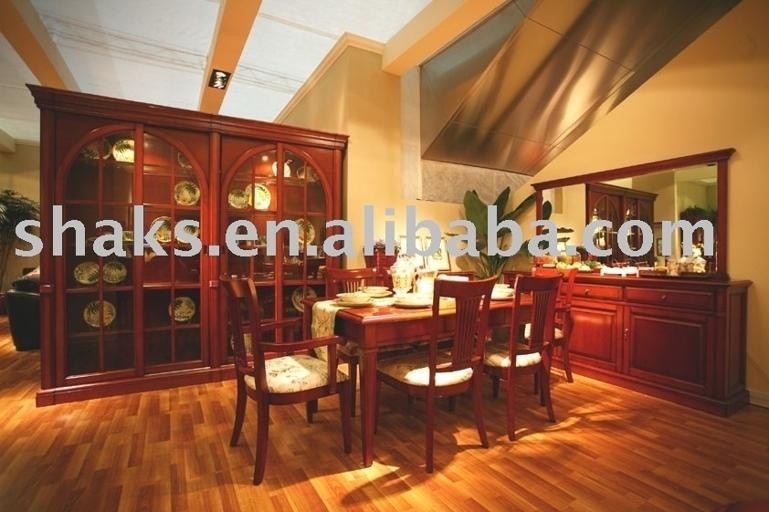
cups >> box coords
[386,263,413,291]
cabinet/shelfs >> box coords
[214,111,350,383]
[585,179,658,268]
[529,276,624,388]
[26,82,214,408]
[624,278,762,420]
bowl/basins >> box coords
[265,262,298,275]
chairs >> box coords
[218,267,573,486]
[6,287,40,352]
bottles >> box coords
[676,254,706,273]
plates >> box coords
[85,299,117,329]
[168,296,196,322]
[175,180,201,206]
[231,330,253,354]
[150,216,201,244]
[489,282,516,301]
[123,231,134,241]
[175,152,193,169]
[270,160,317,181]
[543,263,592,273]
[328,285,430,309]
[295,218,316,244]
[81,136,135,163]
[291,285,317,314]
[228,182,271,209]
[73,262,127,285]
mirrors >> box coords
[531,147,738,276]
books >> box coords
[638,267,667,276]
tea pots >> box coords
[412,269,439,295]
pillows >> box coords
[11,263,40,293]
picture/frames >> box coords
[399,235,426,269]
[424,236,452,272]
